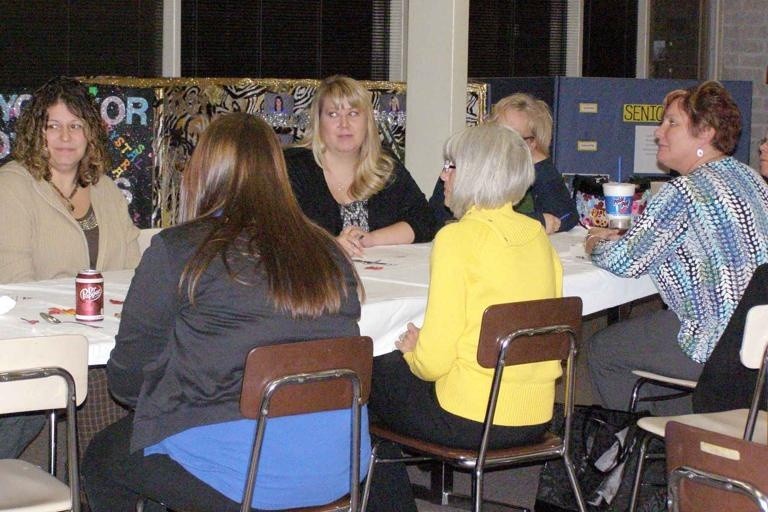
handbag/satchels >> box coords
[537,401,666,512]
[573,175,651,231]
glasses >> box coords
[444,160,455,171]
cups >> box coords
[610,219,631,236]
[602,183,635,229]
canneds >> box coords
[75,269,105,321]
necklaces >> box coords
[331,171,352,194]
[48,179,80,213]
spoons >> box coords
[40,312,103,329]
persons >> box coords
[385,96,403,113]
[74,112,364,511]
[575,78,768,420]
[757,127,768,181]
[362,121,567,510]
[279,75,436,248]
[422,91,581,237]
[268,95,289,112]
[0,76,147,511]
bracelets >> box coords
[583,234,607,248]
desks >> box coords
[357,226,660,315]
[2,269,430,368]
[0,224,659,478]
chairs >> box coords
[664,419,768,510]
[570,263,768,508]
[0,336,86,512]
[116,336,374,512]
[361,297,587,510]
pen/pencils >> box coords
[560,211,574,221]
[41,312,61,323]
[359,234,364,241]
